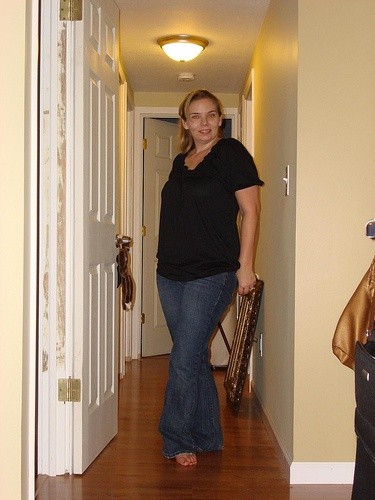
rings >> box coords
[249,287,255,292]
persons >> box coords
[156,89,265,465]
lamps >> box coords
[157,34,209,63]
